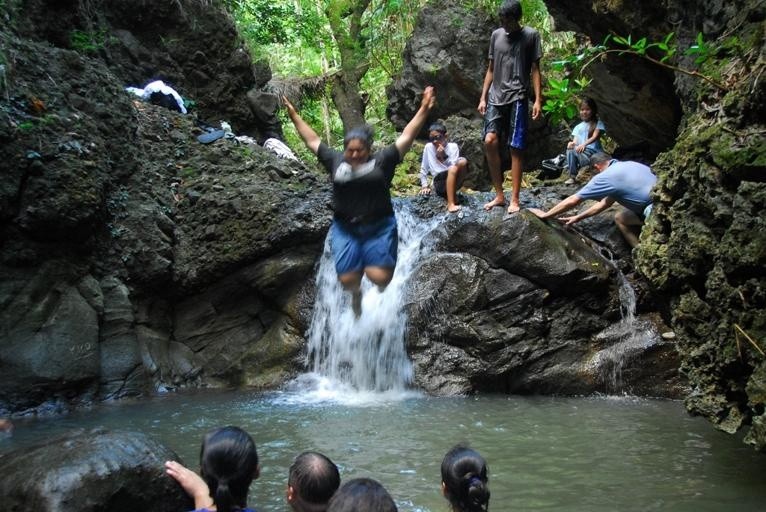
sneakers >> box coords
[564,178,574,184]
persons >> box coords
[476,0,545,214]
[166,459,217,512]
[285,451,340,511]
[525,153,659,249]
[280,85,437,325]
[325,476,400,511]
[59,465,194,512]
[417,122,469,213]
[565,97,606,184]
[197,424,262,511]
[439,443,493,512]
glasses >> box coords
[429,135,441,142]
